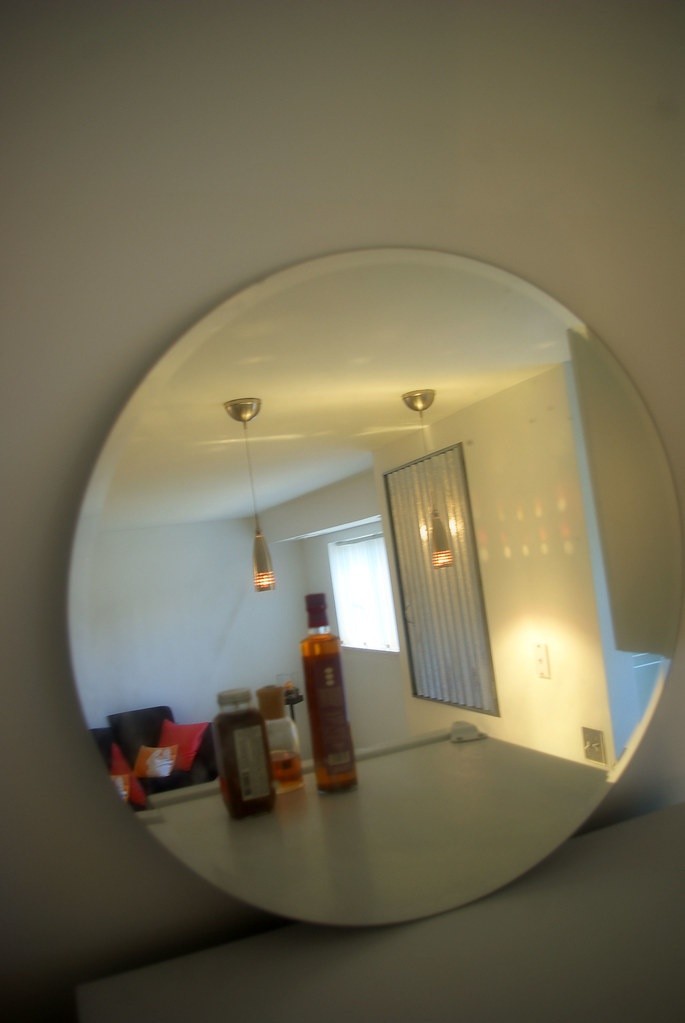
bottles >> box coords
[209,591,359,819]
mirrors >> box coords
[68,245,684,926]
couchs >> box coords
[87,722,221,795]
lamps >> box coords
[223,396,279,592]
[399,388,453,569]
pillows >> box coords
[133,744,179,777]
[108,704,177,764]
[158,718,208,772]
[136,770,186,793]
